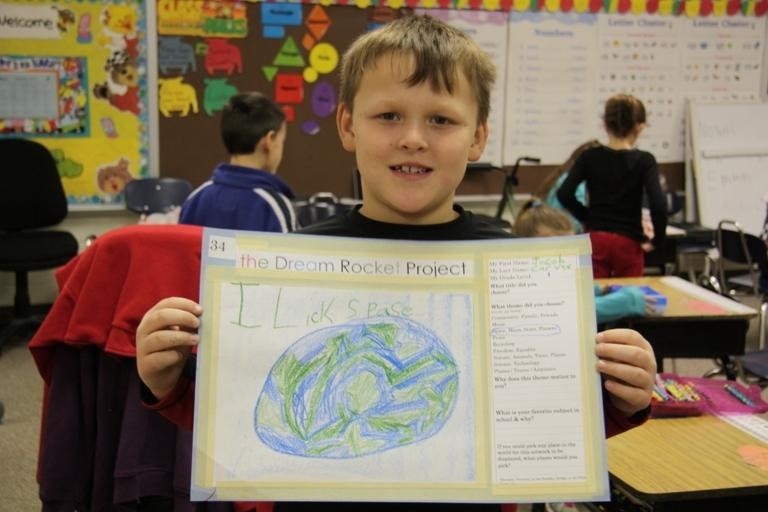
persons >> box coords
[509,197,657,326]
[136,17,658,510]
[548,136,605,233]
[557,94,669,280]
[173,90,297,238]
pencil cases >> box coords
[649,373,768,417]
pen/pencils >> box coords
[724,384,755,407]
[651,373,701,402]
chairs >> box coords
[125,176,191,223]
[0,135,78,347]
[710,220,767,352]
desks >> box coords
[605,410,767,510]
[590,276,758,380]
